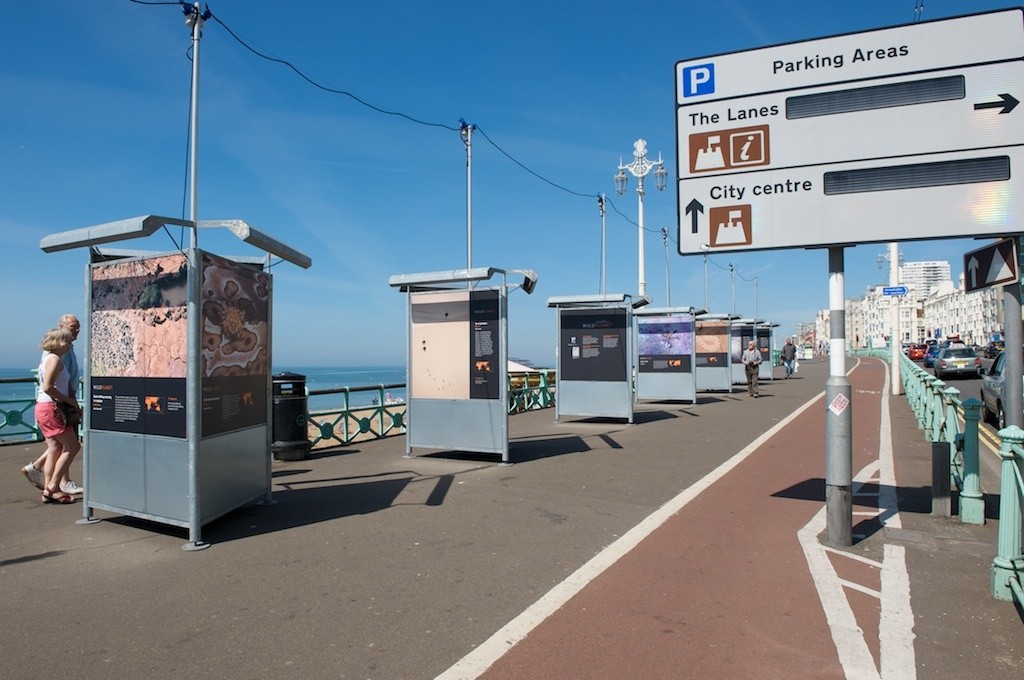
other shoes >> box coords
[753,394,758,398]
[746,393,752,397]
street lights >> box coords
[614,139,669,299]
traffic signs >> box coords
[674,8,1024,257]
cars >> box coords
[927,333,984,378]
[968,339,1024,431]
[902,341,930,360]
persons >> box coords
[372,391,404,405]
[741,341,763,398]
[781,338,797,380]
[818,339,829,361]
[21,313,85,503]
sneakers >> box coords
[59,481,84,494]
[20,462,44,491]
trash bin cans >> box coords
[272,371,311,460]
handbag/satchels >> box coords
[794,361,800,374]
[57,400,80,428]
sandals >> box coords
[41,487,74,503]
